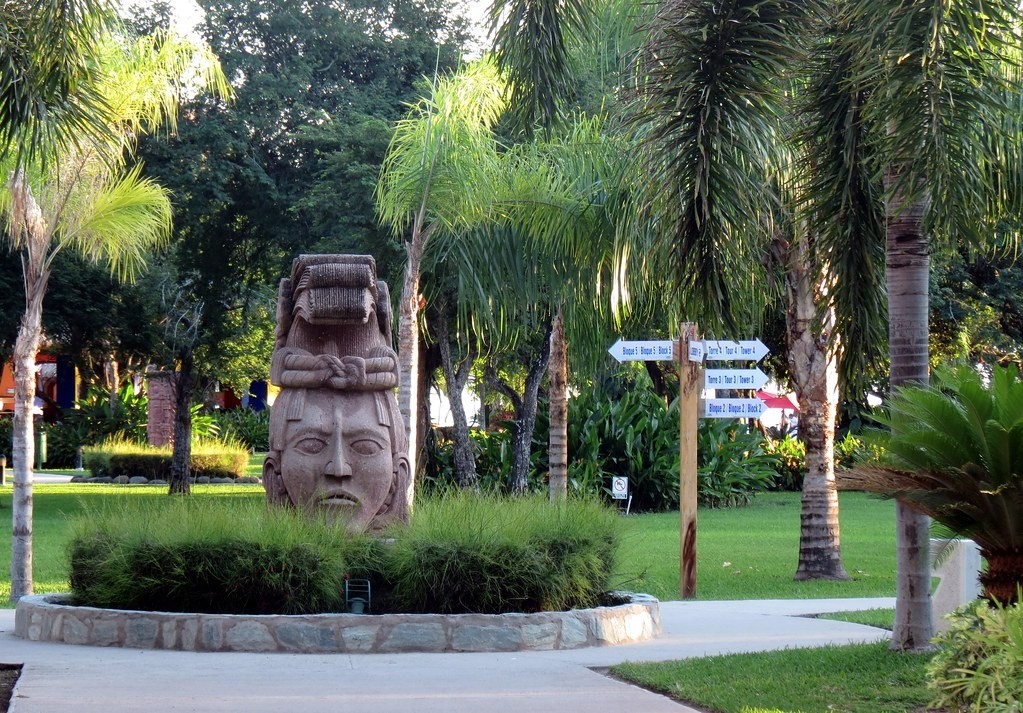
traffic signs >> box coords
[704,395,769,420]
[688,340,709,365]
[704,366,770,392]
[700,337,770,363]
[607,338,674,365]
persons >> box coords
[261,255,410,538]
[782,411,798,433]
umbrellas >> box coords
[755,392,799,438]
